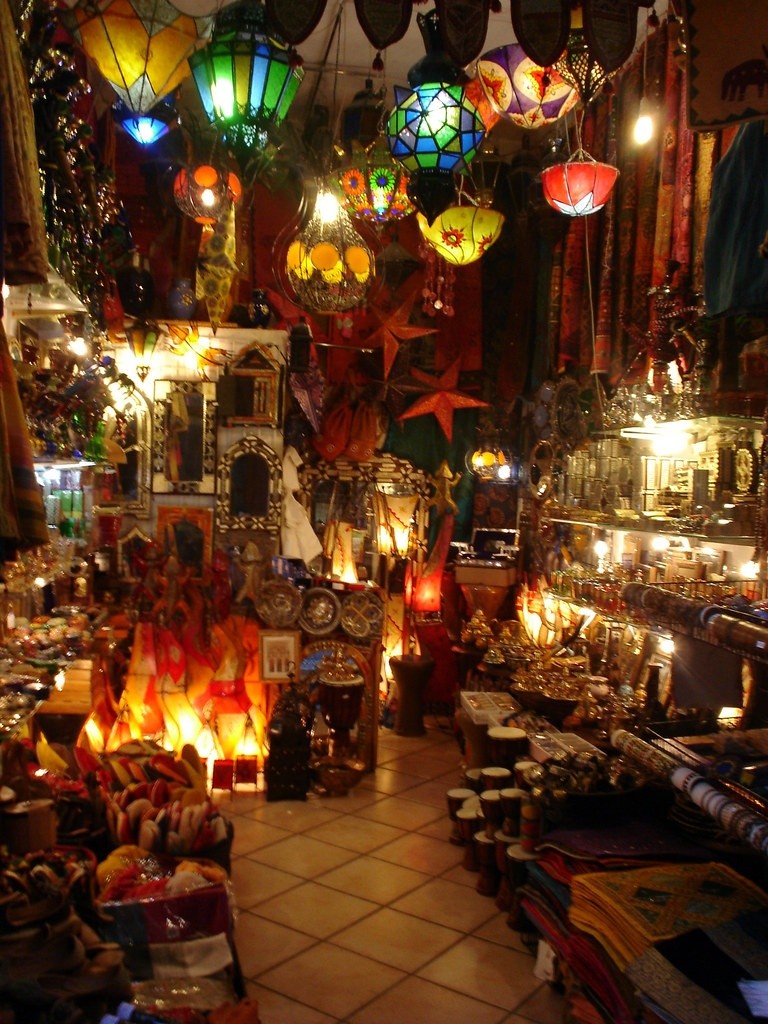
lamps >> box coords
[63,0,620,313]
[462,419,524,485]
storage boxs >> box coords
[455,528,520,585]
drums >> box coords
[499,789,528,838]
[480,767,511,791]
[464,768,485,792]
[462,794,486,833]
[456,808,477,840]
[513,762,538,791]
[447,788,476,822]
[479,789,503,841]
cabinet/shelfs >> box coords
[544,417,768,849]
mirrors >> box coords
[156,505,214,590]
[216,434,284,531]
[224,340,283,434]
[82,376,151,519]
[141,366,219,495]
